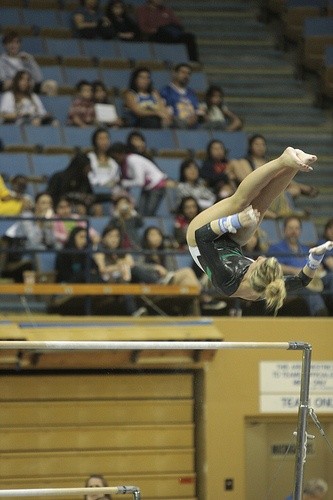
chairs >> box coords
[0,0,333,316]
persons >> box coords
[82,474,112,500]
[186,147,317,310]
[284,477,328,500]
[2,1,333,319]
